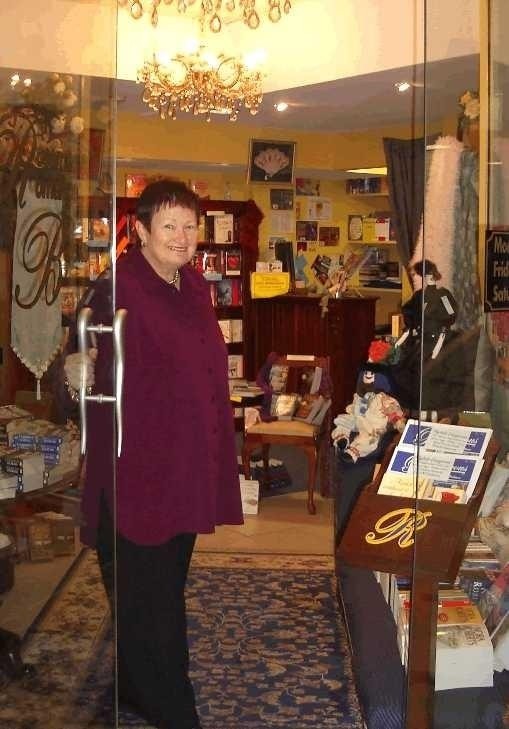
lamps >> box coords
[119,0,292,123]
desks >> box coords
[245,287,380,438]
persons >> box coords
[48,178,244,729]
[380,259,458,408]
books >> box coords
[0,404,72,500]
[189,210,259,399]
[6,510,76,562]
[372,535,509,692]
[267,363,332,425]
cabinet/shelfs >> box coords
[69,197,266,424]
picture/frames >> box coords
[246,136,296,189]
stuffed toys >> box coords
[329,363,407,466]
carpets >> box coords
[0,541,507,725]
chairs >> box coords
[242,353,337,515]
